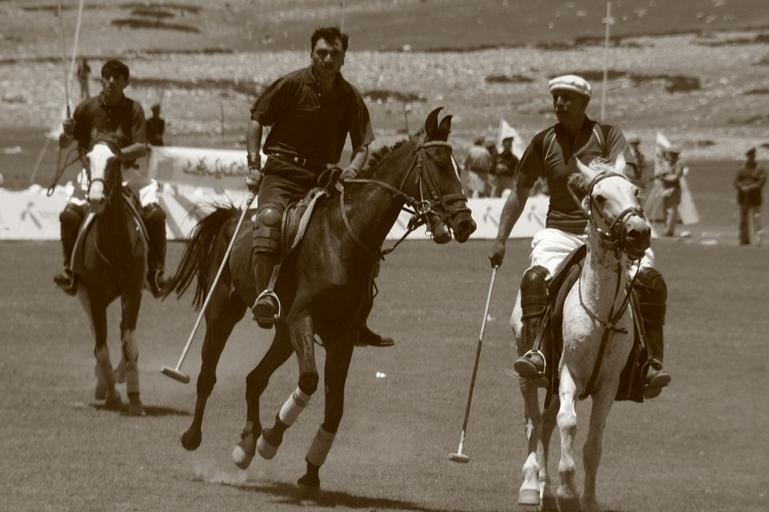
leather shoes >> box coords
[53,269,78,297]
[641,362,670,399]
[269,151,319,170]
[355,327,394,346]
[147,269,177,299]
[251,295,274,330]
[514,346,551,387]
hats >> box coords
[548,74,592,97]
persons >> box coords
[70,57,91,97]
[629,139,644,176]
[48,60,177,299]
[146,104,165,146]
[245,27,395,347]
[459,136,492,198]
[732,149,767,246]
[485,136,500,196]
[496,138,517,195]
[489,74,671,389]
[659,147,682,235]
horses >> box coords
[69,123,151,417]
[510,151,652,512]
[159,107,476,497]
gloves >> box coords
[339,165,359,180]
[246,154,262,189]
